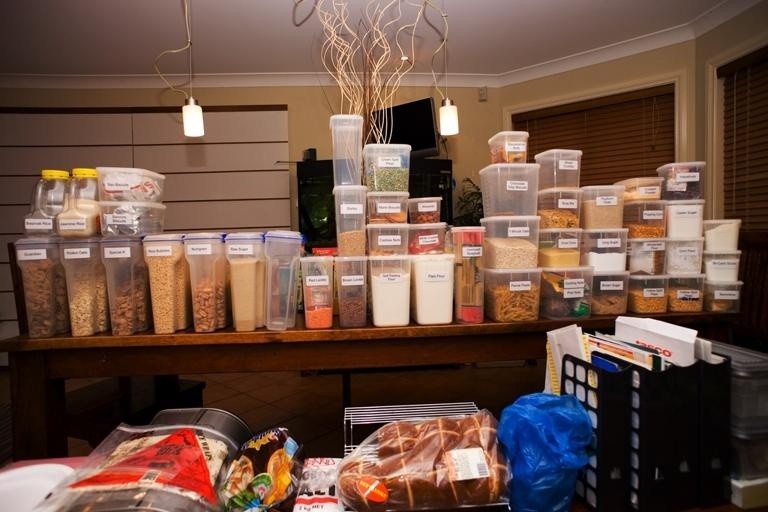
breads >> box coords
[339,412,504,512]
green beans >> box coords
[192,277,226,333]
[144,244,189,334]
[368,168,409,191]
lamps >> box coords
[422,1,460,137]
[152,0,206,139]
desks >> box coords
[3,309,735,470]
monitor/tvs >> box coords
[371,97,440,159]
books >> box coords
[542,314,728,508]
